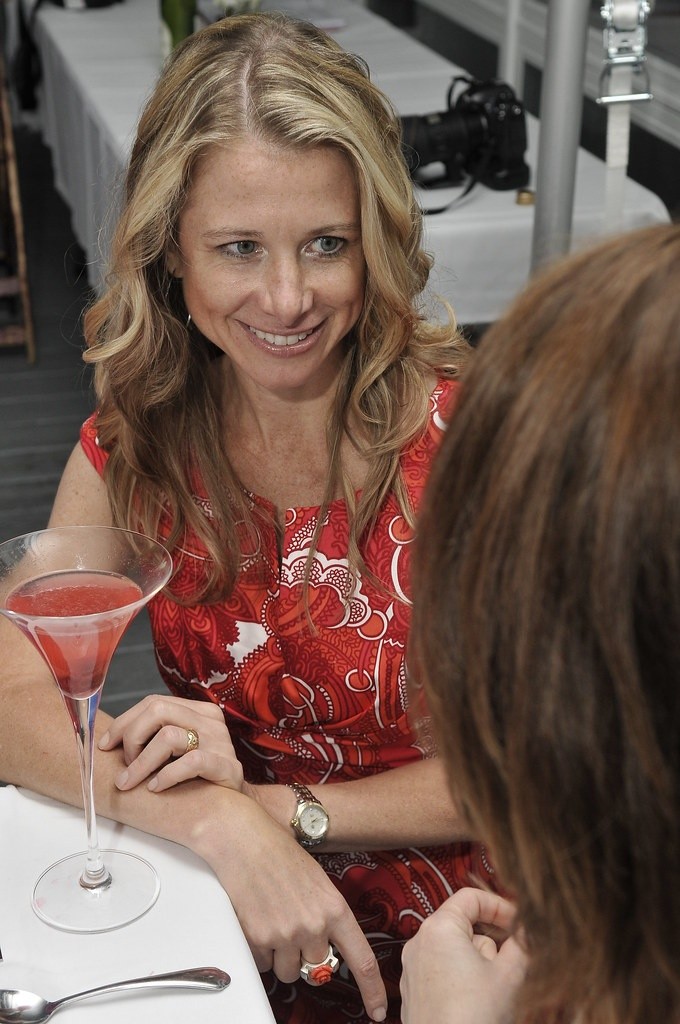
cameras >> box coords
[396,79,531,190]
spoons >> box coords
[0,968,231,1024]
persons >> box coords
[0,15,517,1024]
[400,225,680,1024]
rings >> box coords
[185,729,198,752]
[300,944,339,984]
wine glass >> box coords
[0,527,172,932]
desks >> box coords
[30,0,680,332]
[0,780,279,1024]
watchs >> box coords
[285,782,329,849]
